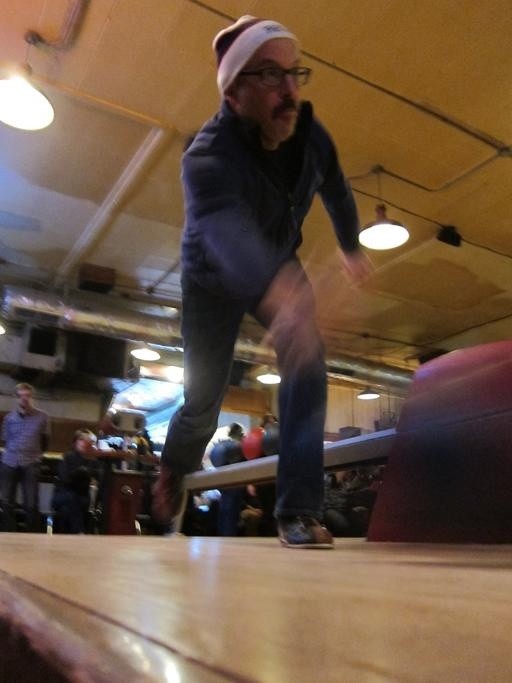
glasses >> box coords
[241,65,313,86]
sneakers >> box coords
[150,469,187,536]
[277,515,335,549]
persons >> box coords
[320,469,372,537]
[152,11,383,552]
[261,427,278,537]
[1,381,53,533]
[226,422,247,443]
[50,426,96,534]
[259,412,275,429]
[219,432,268,531]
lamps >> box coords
[358,165,411,251]
[130,335,162,361]
[0,31,56,131]
[256,360,281,385]
[357,332,381,400]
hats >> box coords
[212,14,301,94]
